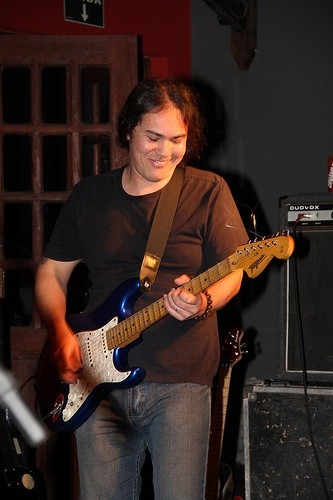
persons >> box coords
[36,78,248,500]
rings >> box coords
[175,306,179,312]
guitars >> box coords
[36,228,294,433]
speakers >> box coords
[279,225,333,386]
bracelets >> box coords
[195,289,212,319]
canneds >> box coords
[327,155,333,195]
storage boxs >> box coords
[241,385,333,500]
